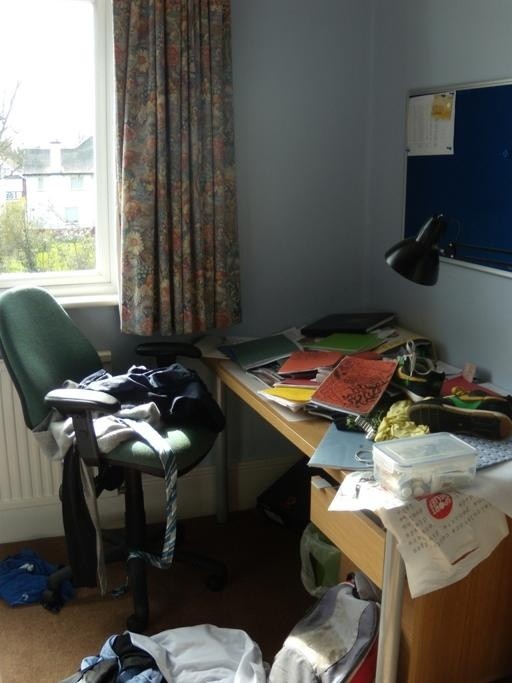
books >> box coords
[197,312,406,469]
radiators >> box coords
[0,350,113,511]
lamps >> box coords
[385,214,511,287]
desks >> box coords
[201,342,511,682]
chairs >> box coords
[0,288,229,631]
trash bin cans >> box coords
[300,522,343,600]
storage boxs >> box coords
[374,433,477,499]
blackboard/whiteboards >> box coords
[400,78,512,279]
